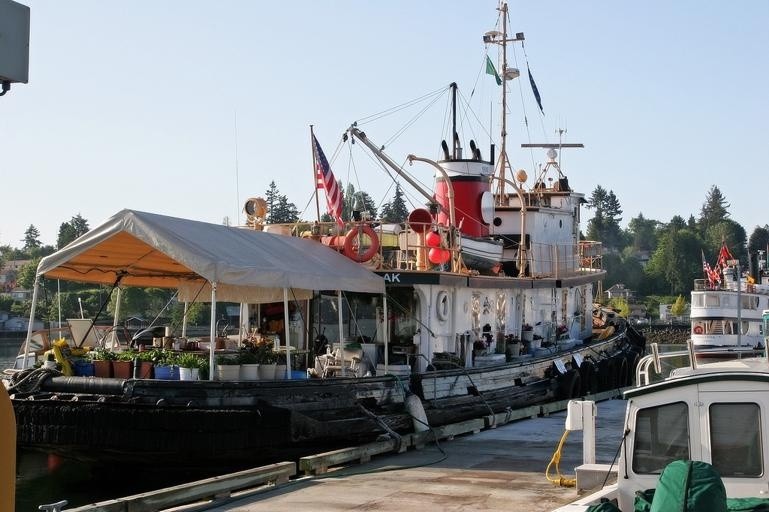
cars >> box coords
[663,316,680,327]
[636,316,649,325]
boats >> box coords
[3,0,650,490]
[606,334,769,512]
[687,240,769,364]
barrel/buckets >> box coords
[404,394,431,433]
[723,267,734,283]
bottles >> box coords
[307,370,311,379]
[274,338,280,350]
[152,326,199,351]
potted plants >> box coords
[71,337,287,382]
[473,323,544,359]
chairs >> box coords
[322,345,363,379]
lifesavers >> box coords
[694,326,703,334]
[436,290,449,320]
[343,224,379,262]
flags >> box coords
[527,67,544,113]
[486,56,502,87]
[312,136,343,228]
[702,244,734,290]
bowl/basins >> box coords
[287,371,306,380]
